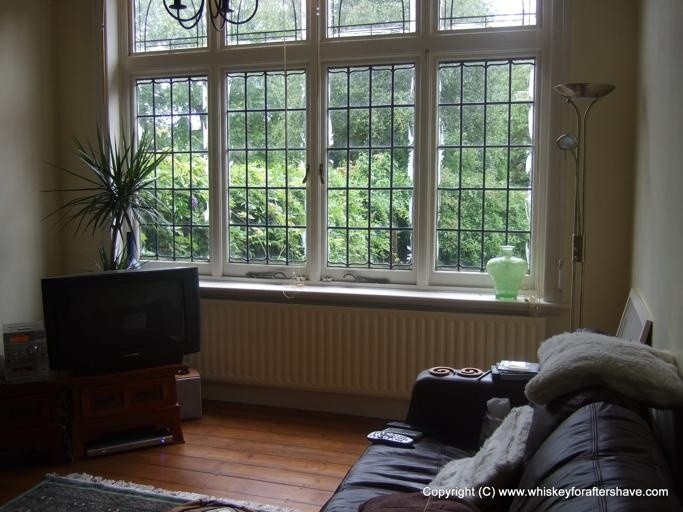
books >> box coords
[491,360,539,381]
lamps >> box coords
[551,81,615,334]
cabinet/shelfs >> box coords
[0,362,188,465]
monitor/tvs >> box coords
[41,267,202,376]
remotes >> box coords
[367,421,421,447]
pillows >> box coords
[356,403,534,512]
[523,326,683,409]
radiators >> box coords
[182,298,547,400]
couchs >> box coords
[320,365,682,512]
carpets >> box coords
[0,470,300,511]
